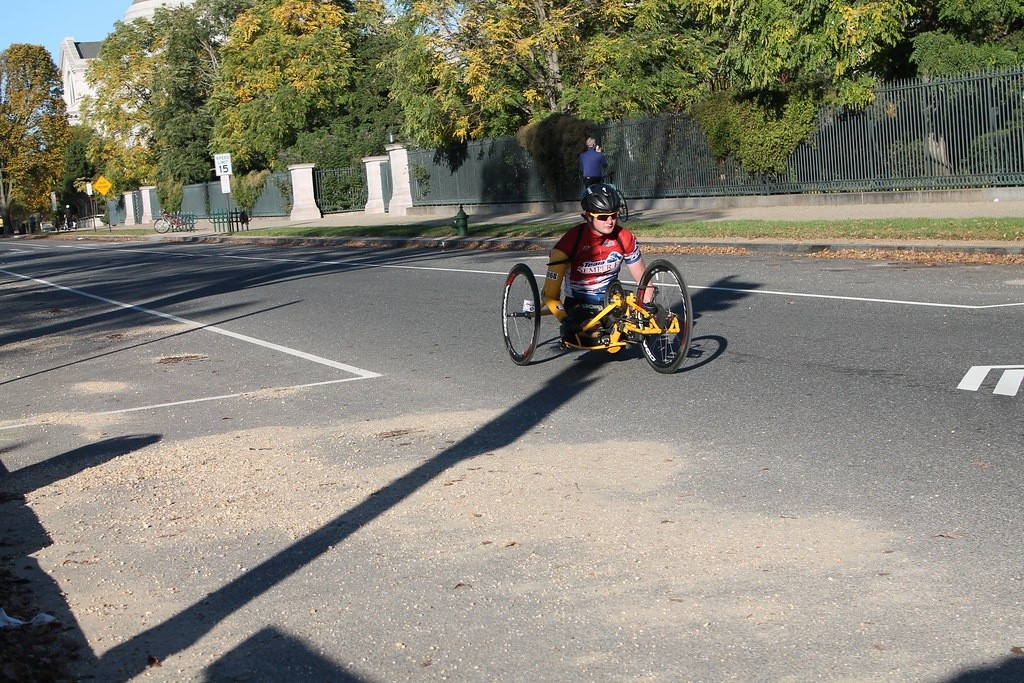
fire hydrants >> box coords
[455,205,471,237]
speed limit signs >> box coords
[214,153,234,176]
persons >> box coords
[64,205,72,230]
[578,137,608,190]
[28,215,35,234]
[0,216,4,234]
[542,184,656,344]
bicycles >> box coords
[599,170,629,223]
[152,208,193,234]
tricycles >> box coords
[500,256,695,374]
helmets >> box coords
[581,184,620,211]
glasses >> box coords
[590,212,620,218]
